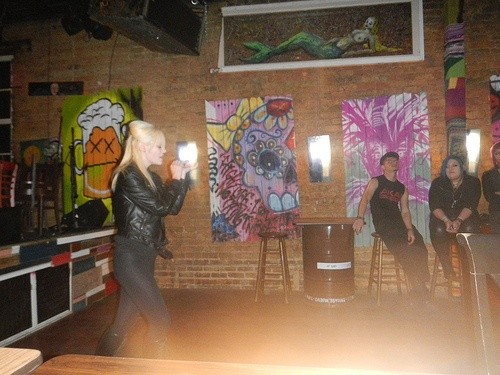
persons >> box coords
[95,120,199,360]
[481,143,500,233]
[351,151,433,321]
[428,155,482,317]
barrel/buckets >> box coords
[302,225,355,308]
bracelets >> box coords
[357,216,364,220]
[407,228,413,232]
[455,217,465,225]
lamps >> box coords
[61,0,112,41]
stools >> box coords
[430,239,464,296]
[255,232,292,304]
[368,233,409,308]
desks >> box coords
[0,347,397,375]
[293,217,366,308]
